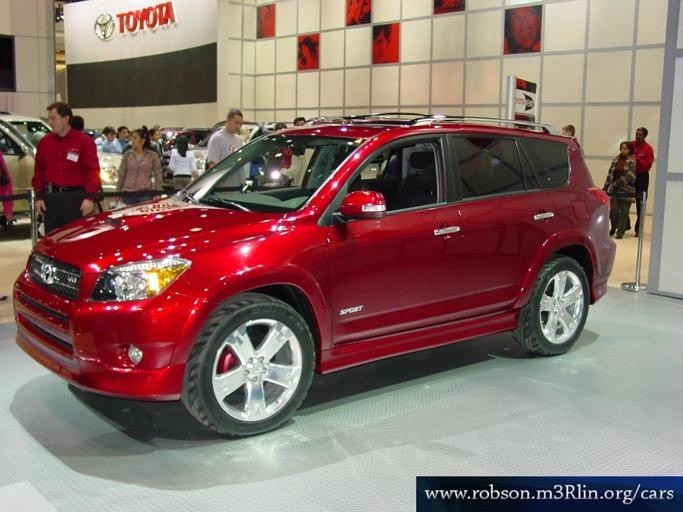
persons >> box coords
[557,124,654,242]
[374,148,402,183]
[1,151,14,307]
[30,102,310,234]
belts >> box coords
[174,174,192,178]
[52,186,78,193]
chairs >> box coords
[391,149,439,209]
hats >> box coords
[637,127,648,136]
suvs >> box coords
[11,111,617,439]
